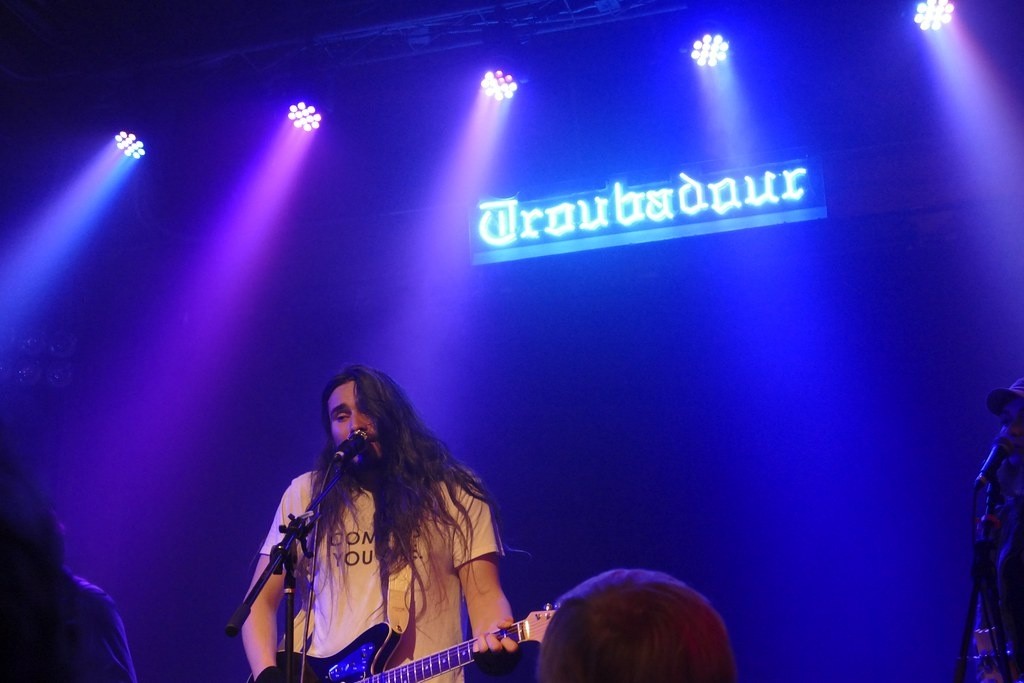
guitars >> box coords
[244,602,560,683]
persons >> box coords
[538,567,736,683]
[0,431,139,683]
[976,378,1024,683]
[240,363,521,683]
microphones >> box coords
[974,437,1013,491]
[334,429,367,463]
